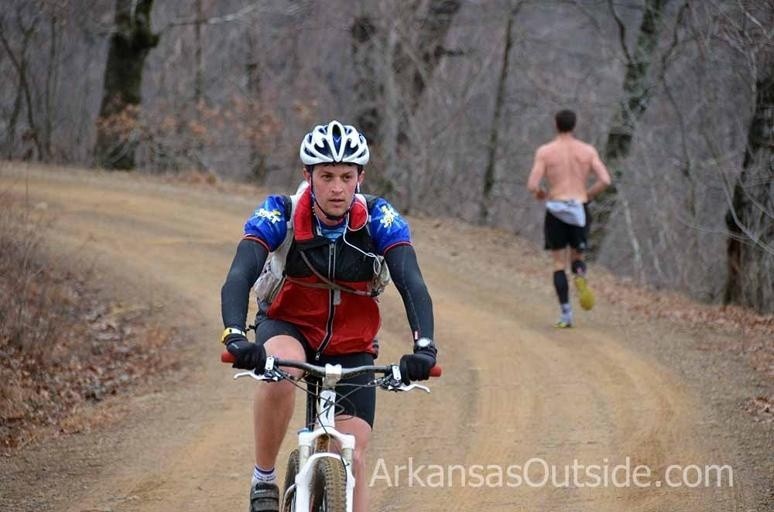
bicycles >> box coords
[221,351,441,512]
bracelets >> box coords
[221,327,246,343]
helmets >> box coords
[299,120,370,165]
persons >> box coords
[220,122,438,511]
[525,109,611,329]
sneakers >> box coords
[573,275,595,310]
[249,481,280,512]
[551,319,572,329]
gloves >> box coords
[224,333,266,375]
[399,343,437,386]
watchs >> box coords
[412,336,437,354]
